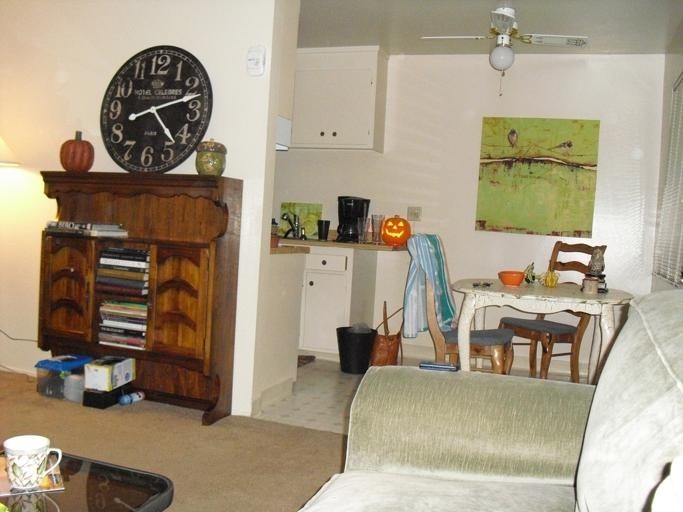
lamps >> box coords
[488,36,514,72]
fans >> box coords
[420,8,588,51]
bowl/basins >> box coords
[498,271,525,287]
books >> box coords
[45,220,123,231]
[94,244,149,352]
[44,228,128,238]
[0,454,66,498]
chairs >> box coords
[299,285,683,509]
[404,233,607,384]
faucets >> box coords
[282,211,300,239]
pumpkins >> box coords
[59,130,94,172]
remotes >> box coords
[419,361,459,372]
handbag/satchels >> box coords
[371,330,399,365]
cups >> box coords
[317,219,331,242]
[372,214,384,243]
[3,435,63,490]
[357,217,370,243]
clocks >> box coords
[100,45,211,173]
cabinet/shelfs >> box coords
[298,246,354,362]
[289,46,389,154]
[36,171,243,425]
